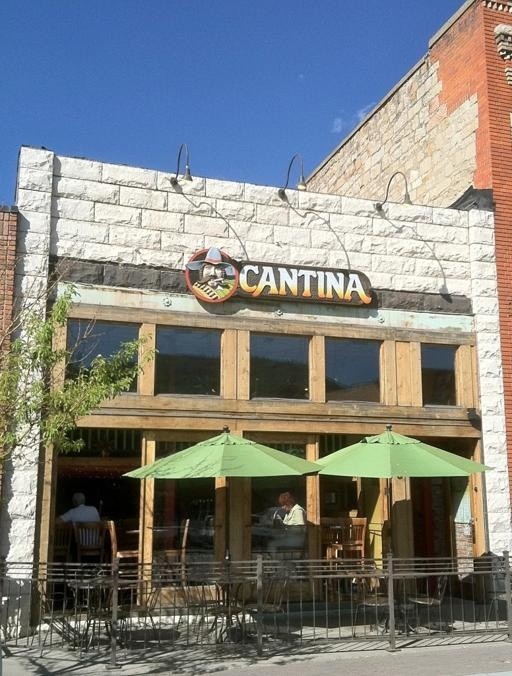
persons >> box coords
[194,248,234,299]
[56,492,103,547]
[266,493,305,562]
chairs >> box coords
[322,517,367,573]
[55,519,139,623]
[147,520,192,609]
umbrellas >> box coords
[313,421,494,556]
[121,422,318,559]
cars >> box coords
[125,508,296,550]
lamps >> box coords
[374,166,413,213]
[167,143,193,185]
[277,149,308,198]
